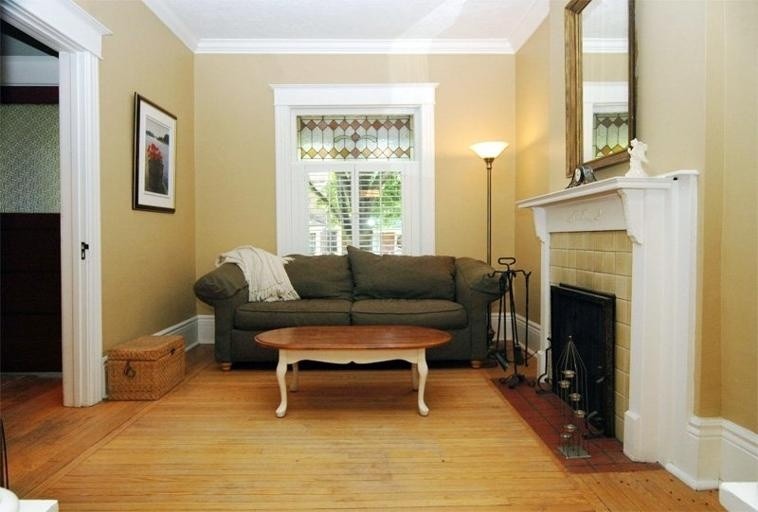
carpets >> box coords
[492,377,661,473]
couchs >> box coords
[194,253,508,371]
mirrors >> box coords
[565,1,637,177]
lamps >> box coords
[471,141,510,266]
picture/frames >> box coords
[131,93,177,213]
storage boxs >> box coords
[108,335,186,401]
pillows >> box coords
[282,245,456,302]
